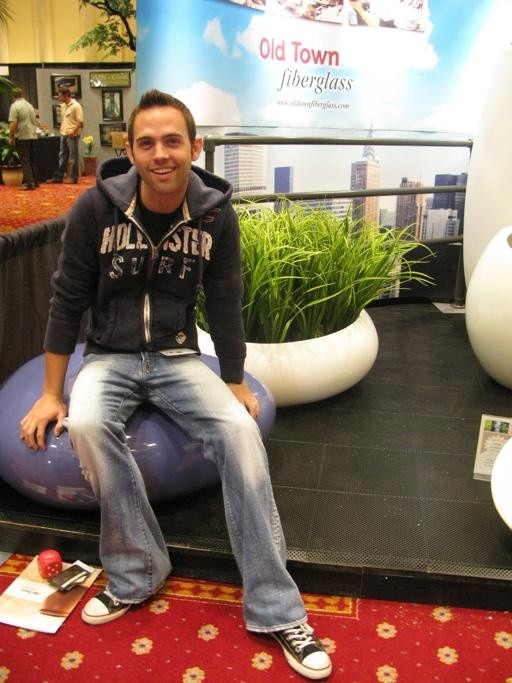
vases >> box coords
[83,155,96,175]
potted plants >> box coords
[189,190,440,410]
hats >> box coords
[12,88,22,96]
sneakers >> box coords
[46,177,56,182]
[19,183,38,189]
[80,588,131,625]
[64,179,75,184]
[268,621,332,680]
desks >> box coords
[21,135,61,184]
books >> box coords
[472,411,512,484]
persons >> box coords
[20,89,332,680]
[46,89,84,184]
[8,88,39,190]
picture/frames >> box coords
[99,122,127,146]
[102,89,124,120]
[52,104,62,127]
[90,70,131,87]
[50,73,82,98]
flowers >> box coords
[81,135,96,155]
